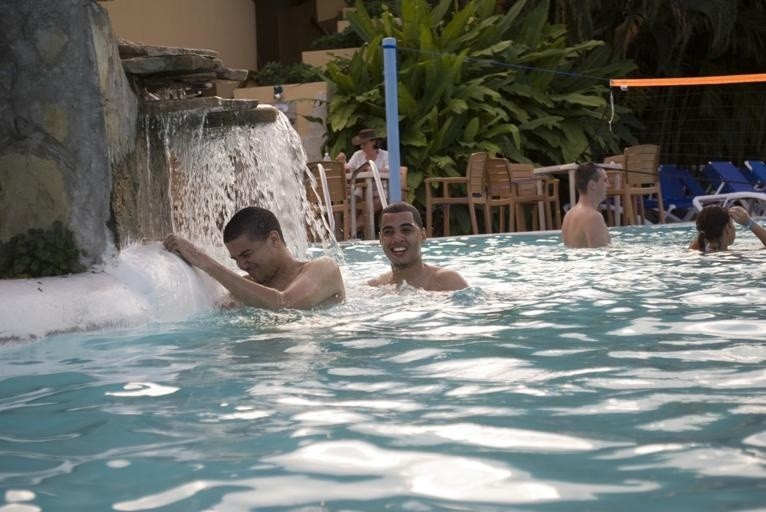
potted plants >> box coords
[233,23,369,104]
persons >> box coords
[335,130,388,236]
[562,162,610,248]
[163,207,345,311]
[368,201,469,291]
[688,204,766,252]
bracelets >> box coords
[746,219,753,230]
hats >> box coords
[351,128,386,146]
